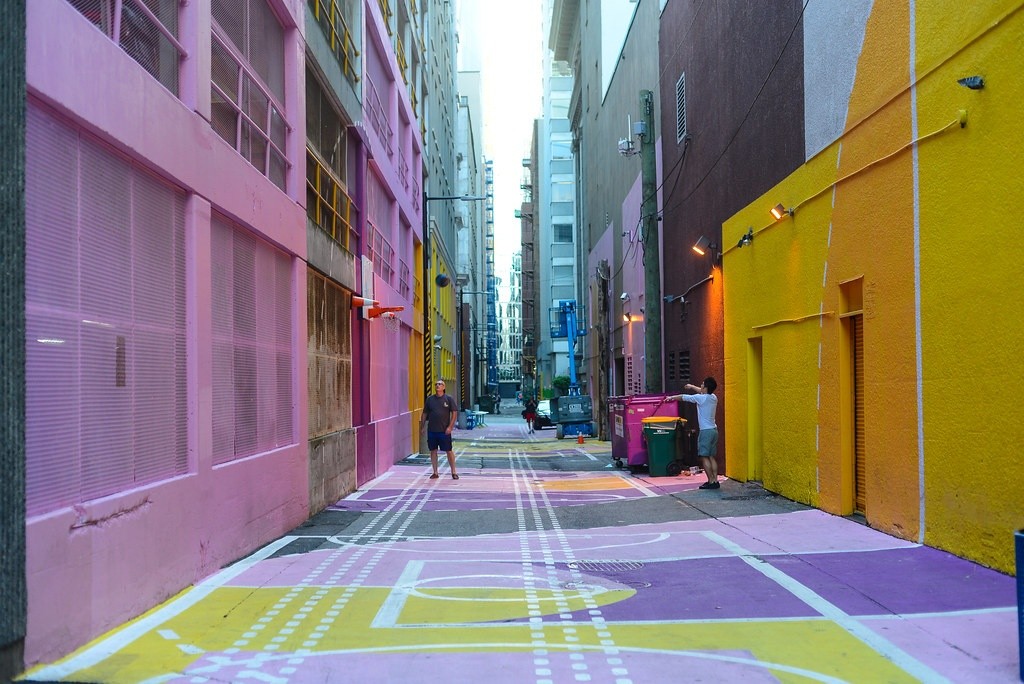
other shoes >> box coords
[452,474,459,480]
[699,481,716,489]
[715,481,720,488]
[528,431,531,434]
[430,473,439,478]
[532,429,534,433]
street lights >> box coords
[460,288,487,412]
[472,321,498,406]
[420,192,487,421]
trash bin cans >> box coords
[606,391,678,475]
[641,416,688,477]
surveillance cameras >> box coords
[620,293,628,301]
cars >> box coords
[532,400,554,430]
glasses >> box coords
[434,382,444,386]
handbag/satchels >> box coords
[521,410,526,415]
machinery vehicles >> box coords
[548,301,598,440]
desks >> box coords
[473,411,489,428]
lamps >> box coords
[690,234,720,267]
[770,202,797,221]
[622,310,634,323]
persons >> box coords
[421,380,459,479]
[492,394,501,414]
[663,377,721,489]
[525,396,537,434]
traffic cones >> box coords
[373,312,395,319]
[576,430,586,444]
[351,292,380,310]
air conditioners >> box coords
[523,333,535,346]
